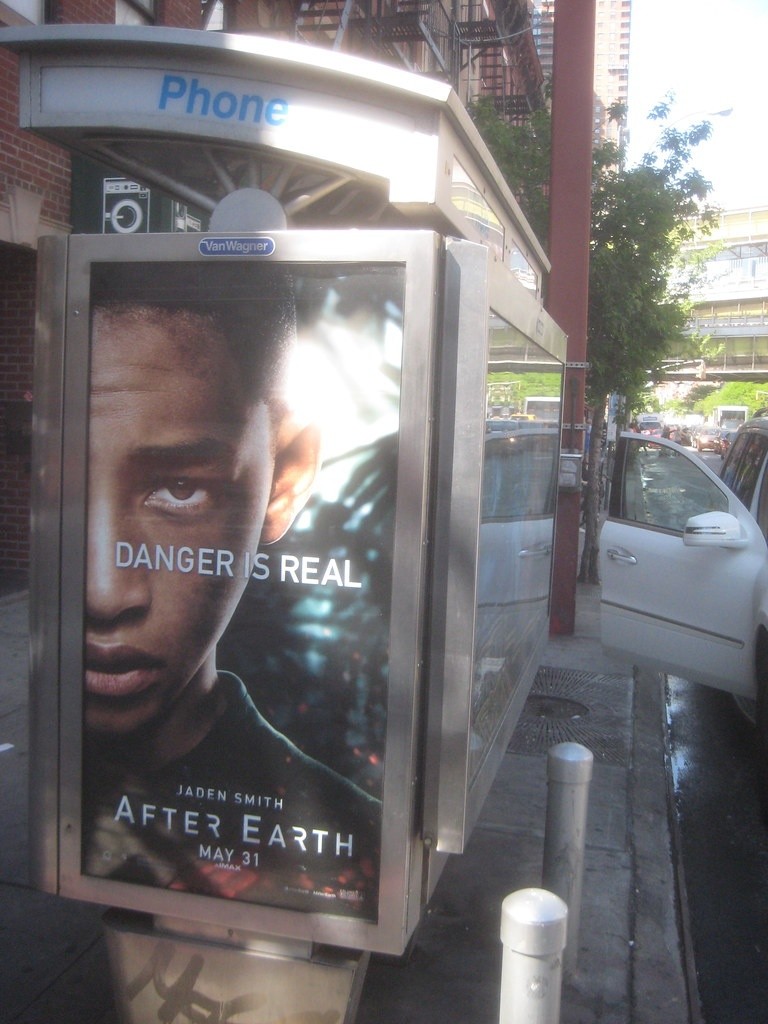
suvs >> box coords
[599,408,768,745]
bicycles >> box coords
[578,462,611,528]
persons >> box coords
[85,260,381,927]
[584,417,593,464]
[659,426,687,458]
[627,423,641,451]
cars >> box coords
[637,414,737,461]
[510,415,536,421]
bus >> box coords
[713,406,747,430]
[524,395,560,420]
